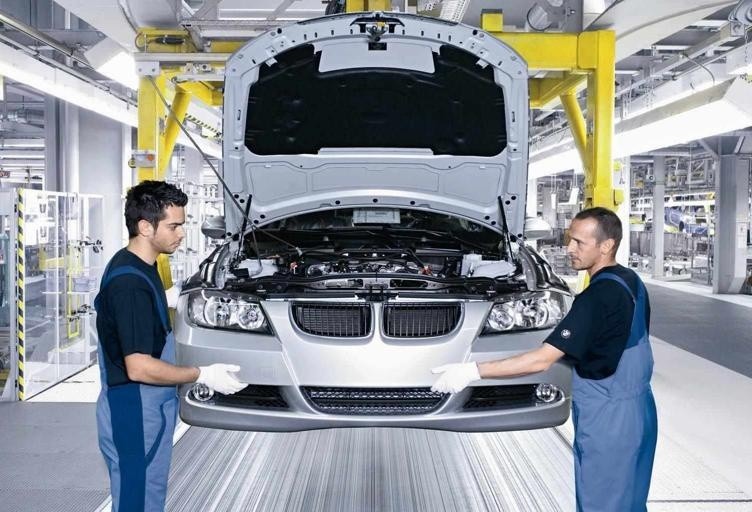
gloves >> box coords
[196,364,249,396]
[431,361,482,395]
[165,280,182,309]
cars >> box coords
[173,12,577,432]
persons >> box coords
[429,207,658,512]
[93,180,248,512]
